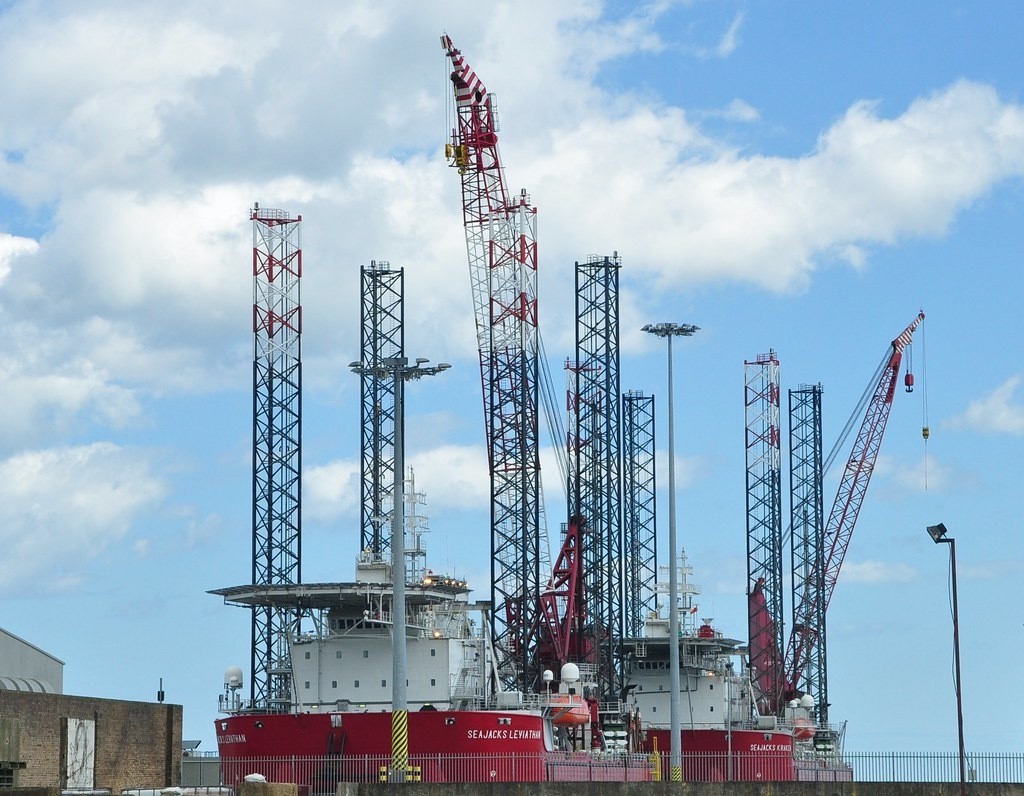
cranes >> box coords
[246,30,929,754]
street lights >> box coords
[926,522,967,796]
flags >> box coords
[690,607,698,614]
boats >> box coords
[213,463,855,786]
